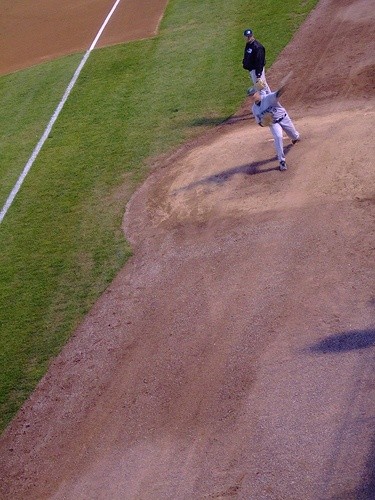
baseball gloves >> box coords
[254,77,265,92]
[259,113,273,128]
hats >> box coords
[247,87,257,96]
[243,30,252,37]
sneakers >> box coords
[291,139,297,145]
[279,160,288,171]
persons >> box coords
[247,84,299,171]
[242,29,271,96]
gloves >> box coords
[255,71,261,78]
